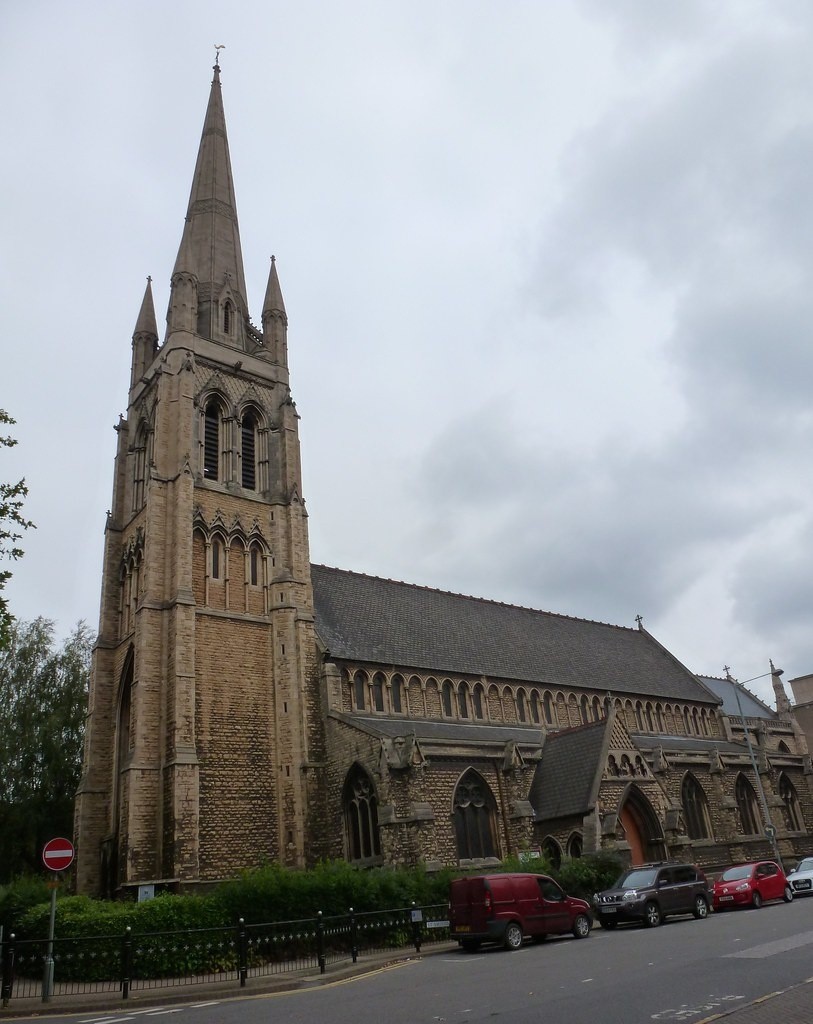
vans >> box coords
[449,873,593,951]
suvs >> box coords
[594,861,713,928]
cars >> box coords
[711,861,793,911]
[786,857,813,896]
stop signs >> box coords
[43,837,75,871]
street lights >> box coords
[733,668,787,877]
